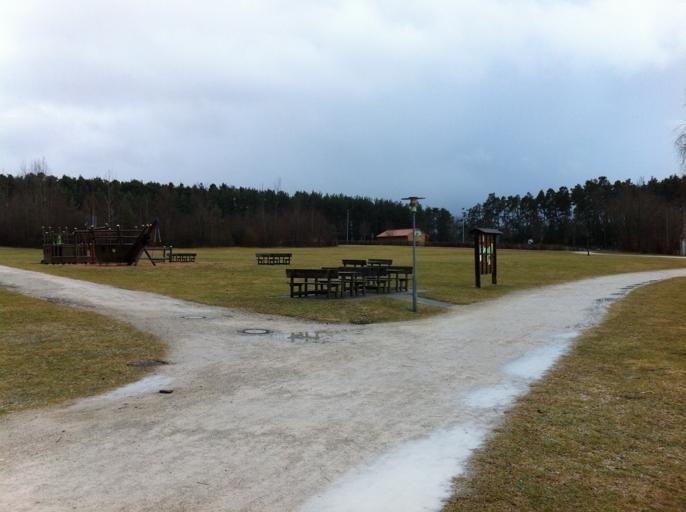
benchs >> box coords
[286,259,413,298]
